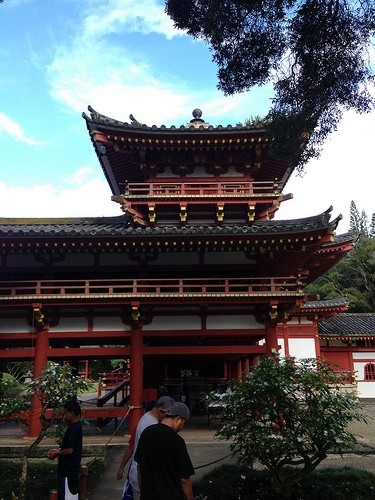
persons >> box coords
[134,402,195,500]
[116,401,157,500]
[48,400,83,500]
[129,396,175,500]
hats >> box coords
[156,396,175,413]
[165,402,190,422]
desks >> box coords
[208,403,236,426]
